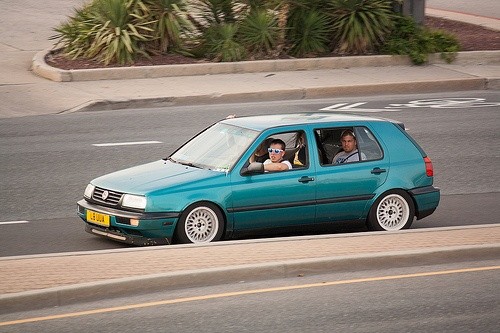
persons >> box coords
[331,129,367,166]
[293,132,329,166]
[263,139,292,173]
[248,138,270,169]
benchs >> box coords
[322,143,345,164]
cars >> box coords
[74,112,440,245]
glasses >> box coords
[268,148,283,154]
